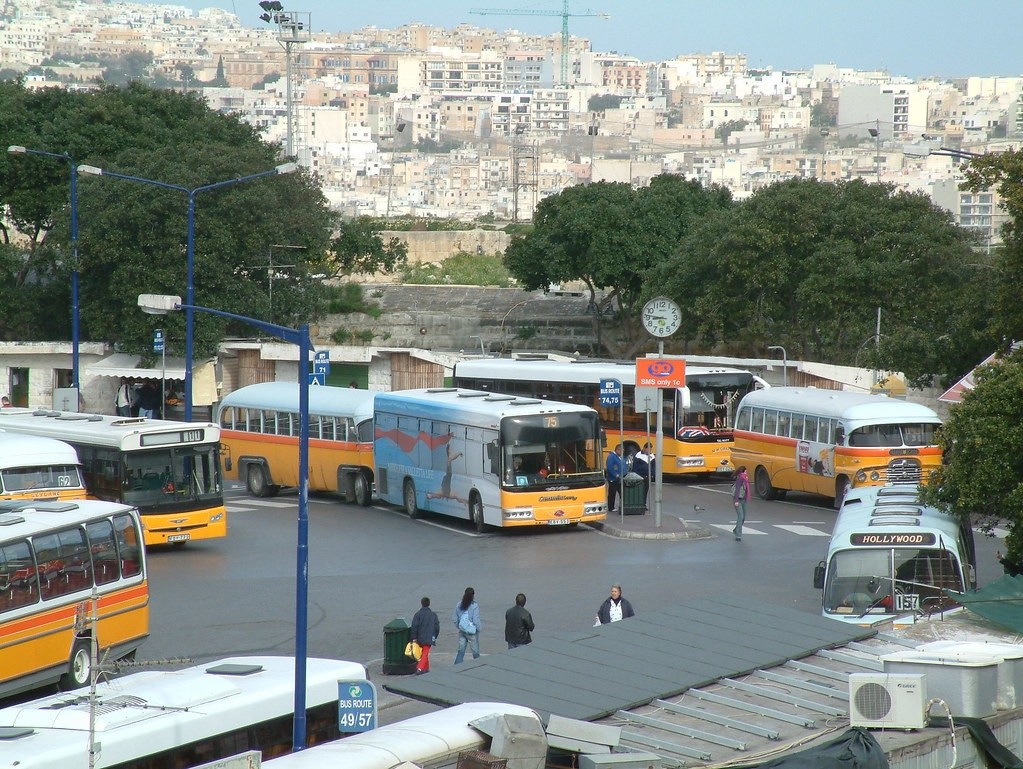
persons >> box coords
[996,535,1013,574]
[349,382,358,389]
[505,594,534,649]
[66,372,73,383]
[731,466,751,542]
[598,584,634,624]
[2,396,11,408]
[633,442,655,511]
[808,458,828,475]
[425,444,468,504]
[410,597,439,675]
[115,378,160,419]
[454,587,480,663]
[606,444,626,512]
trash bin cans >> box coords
[384,620,418,675]
[619,473,648,515]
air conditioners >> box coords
[849,672,928,729]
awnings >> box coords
[86,353,212,380]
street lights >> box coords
[768,345,787,388]
[259,0,312,157]
[10,144,85,390]
[136,292,311,753]
[78,156,299,496]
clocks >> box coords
[641,297,682,337]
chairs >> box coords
[0,544,139,612]
[141,472,162,492]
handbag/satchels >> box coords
[731,485,745,498]
[458,618,476,634]
[405,642,422,660]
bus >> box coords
[0,654,368,769]
[733,386,955,511]
[0,406,228,546]
[1,433,87,504]
[215,380,388,509]
[372,389,611,534]
[451,351,772,483]
[261,694,546,769]
[0,500,154,700]
[812,481,980,633]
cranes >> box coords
[465,1,611,87]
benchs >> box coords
[228,418,342,440]
